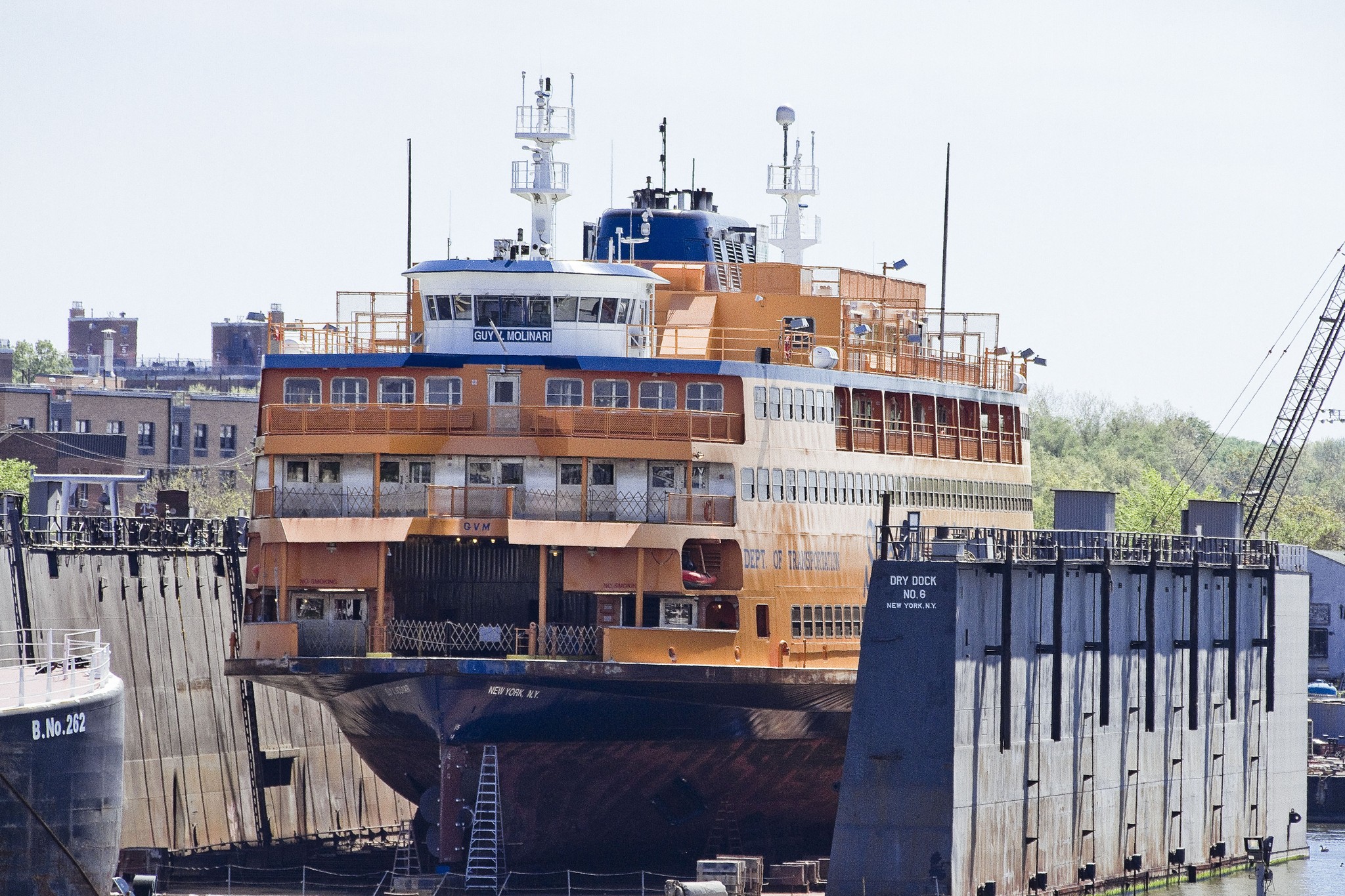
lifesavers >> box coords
[703,501,716,523]
[783,336,793,358]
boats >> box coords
[0,494,124,896]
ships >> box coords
[223,63,1049,830]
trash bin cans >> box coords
[435,862,455,875]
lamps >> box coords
[855,325,872,336]
[776,105,796,131]
[1020,348,1035,358]
[893,259,908,271]
[994,347,1009,356]
[908,335,921,342]
[791,318,810,329]
[539,244,552,255]
[1034,357,1047,366]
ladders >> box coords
[390,819,421,892]
[463,744,507,896]
